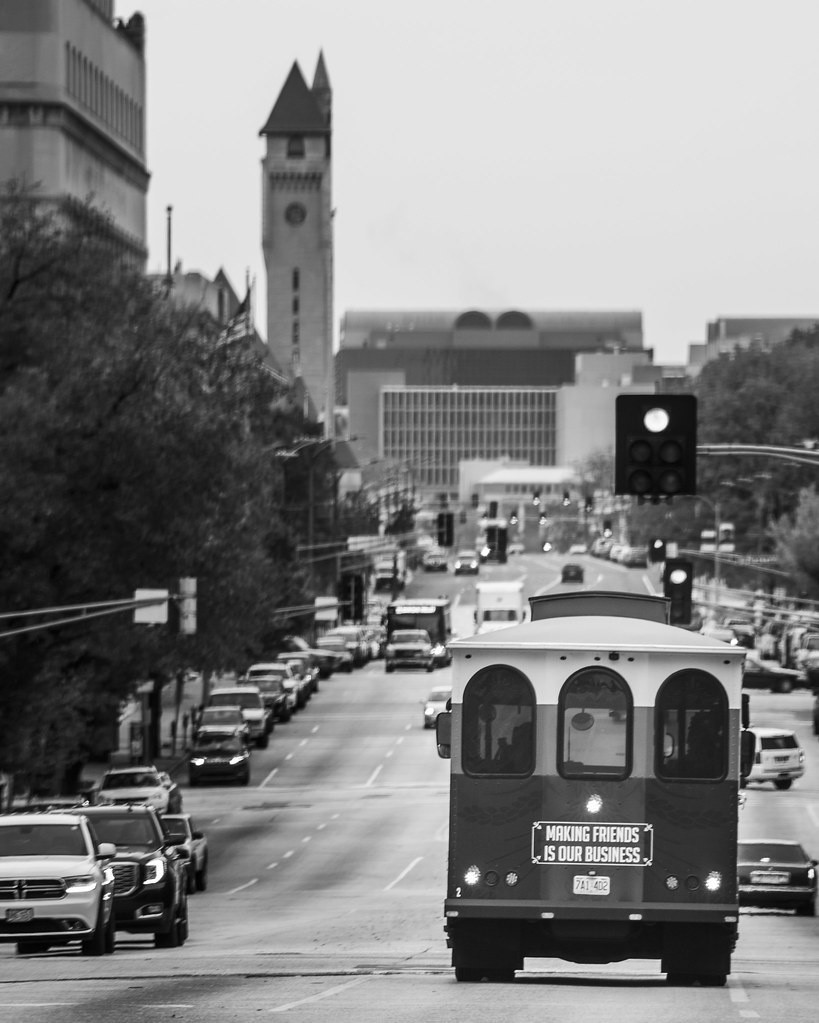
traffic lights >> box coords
[662,558,696,623]
[611,391,699,499]
[649,537,668,563]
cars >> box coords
[139,812,210,894]
[588,536,647,567]
[698,605,819,732]
[186,579,529,789]
[560,563,584,583]
[456,550,480,574]
[422,551,448,571]
[737,837,819,917]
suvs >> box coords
[92,766,184,817]
[32,800,193,948]
[0,809,122,957]
[741,727,806,792]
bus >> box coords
[429,614,755,987]
[527,559,705,634]
